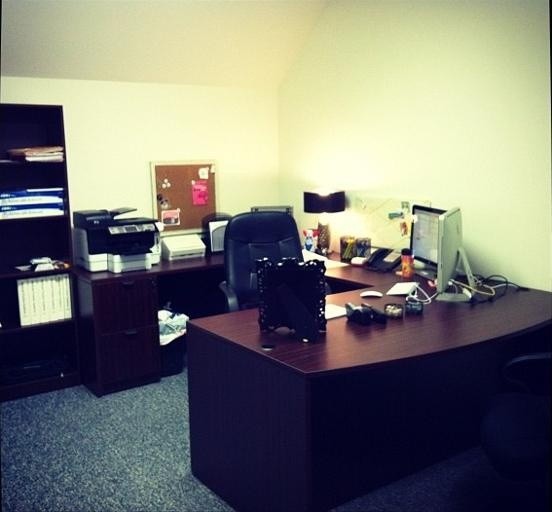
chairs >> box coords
[464,343,552,484]
[211,203,307,316]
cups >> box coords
[383,303,403,318]
[405,300,423,313]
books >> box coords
[8,146,64,163]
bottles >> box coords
[400,248,413,280]
[304,230,315,252]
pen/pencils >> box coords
[341,236,356,260]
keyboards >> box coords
[385,281,417,296]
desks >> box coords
[64,243,423,400]
[183,275,551,512]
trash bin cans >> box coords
[158,313,189,377]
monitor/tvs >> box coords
[435,206,476,302]
[409,203,448,281]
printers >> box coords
[71,205,163,273]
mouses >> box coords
[360,290,383,298]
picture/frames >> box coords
[247,252,331,347]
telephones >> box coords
[367,248,401,272]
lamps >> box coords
[298,187,349,216]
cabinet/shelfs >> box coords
[1,102,87,406]
